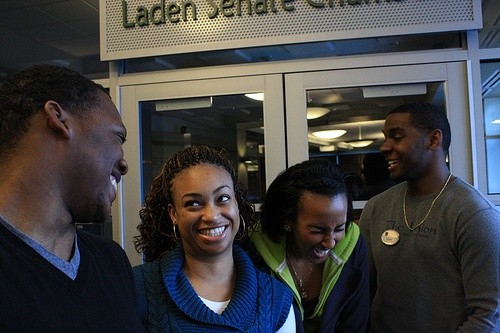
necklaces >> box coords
[286,251,314,298]
[403,174,452,231]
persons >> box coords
[0,65,150,333]
[120,145,299,333]
[242,158,377,333]
[355,103,500,333]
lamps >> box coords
[313,130,347,138]
[345,139,377,147]
[306,106,329,119]
